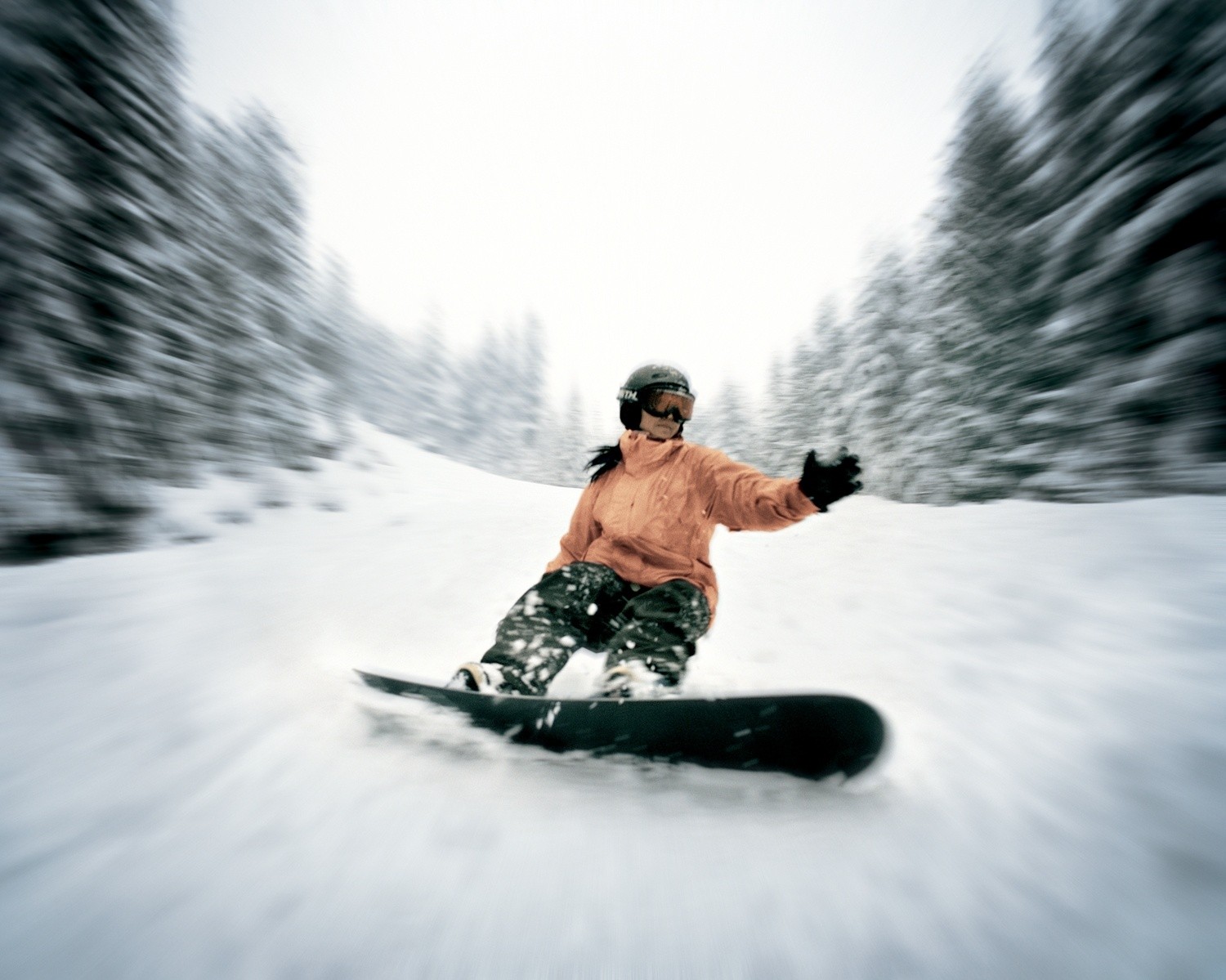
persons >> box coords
[457,364,865,698]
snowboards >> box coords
[352,661,889,785]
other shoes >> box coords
[599,686,633,698]
[465,664,546,696]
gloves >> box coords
[798,444,864,512]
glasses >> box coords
[641,389,695,423]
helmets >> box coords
[619,365,689,405]
